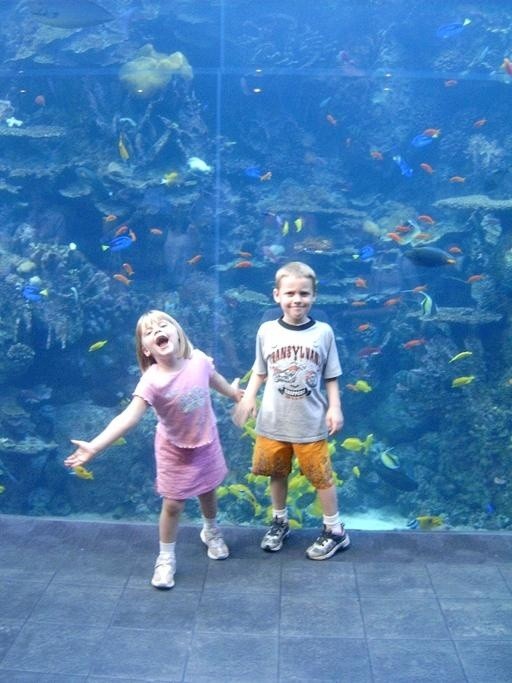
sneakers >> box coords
[261,519,290,551]
[200,528,230,559]
[151,557,175,587]
[305,526,350,560]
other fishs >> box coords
[0,1,512,537]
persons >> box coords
[231,261,352,562]
[63,310,245,589]
